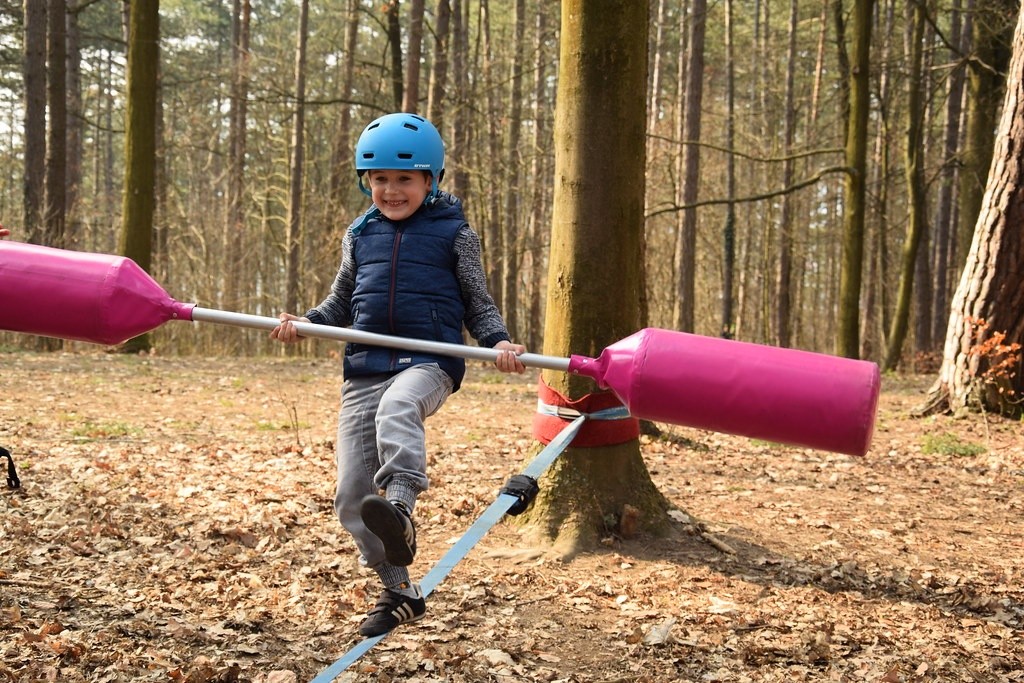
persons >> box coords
[268,112,527,635]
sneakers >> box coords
[360,494,416,567]
[359,584,426,635]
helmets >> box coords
[355,112,445,183]
